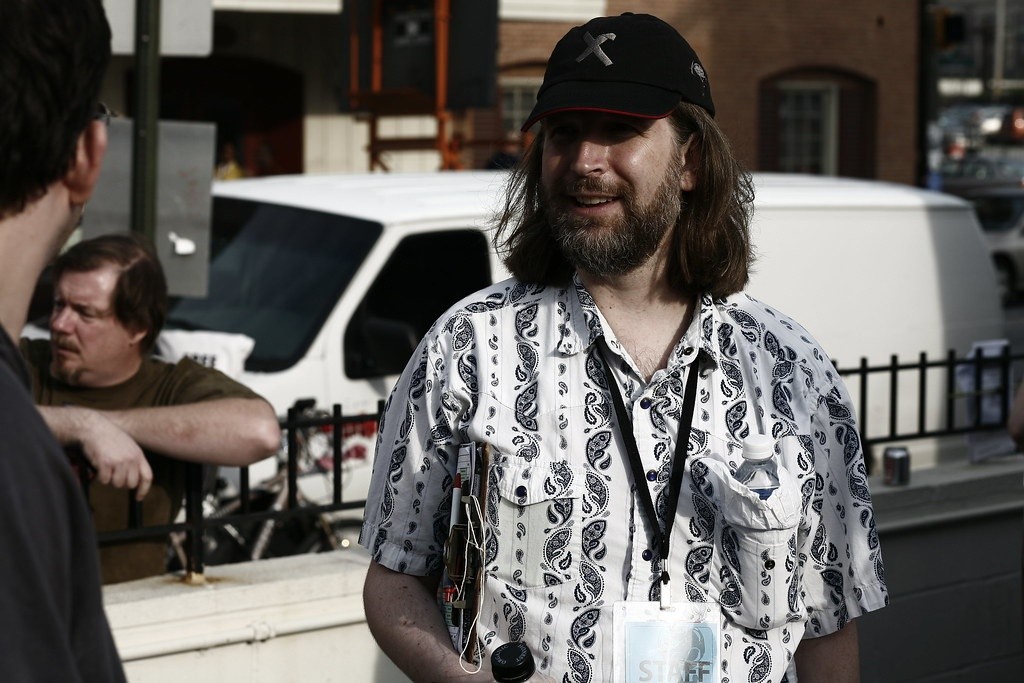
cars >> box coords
[931,105,1024,212]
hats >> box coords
[521,14,716,136]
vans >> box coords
[26,165,1006,549]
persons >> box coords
[18,233,288,589]
[355,11,894,682]
[1,2,135,682]
